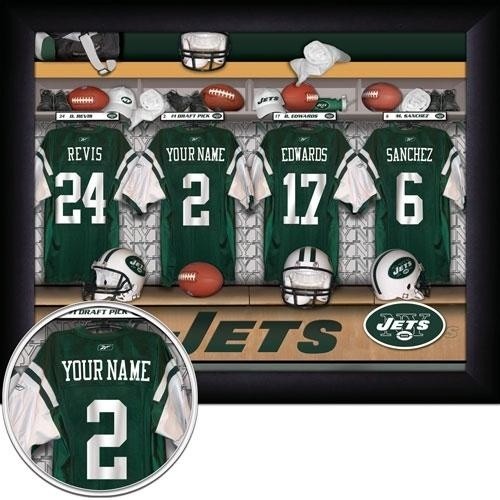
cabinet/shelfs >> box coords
[34,59,466,123]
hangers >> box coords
[284,119,319,133]
[69,121,101,135]
[64,318,132,341]
[392,118,424,136]
[178,118,209,137]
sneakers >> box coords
[425,88,442,112]
[440,88,461,113]
[187,89,211,114]
[37,87,54,112]
[53,90,74,114]
[164,89,194,113]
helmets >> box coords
[78,245,148,305]
[279,243,335,312]
[368,245,432,303]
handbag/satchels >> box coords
[32,29,123,77]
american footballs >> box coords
[201,84,243,110]
[283,81,317,111]
[362,82,402,112]
[67,86,109,110]
[179,263,223,297]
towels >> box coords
[289,40,352,86]
[394,87,431,113]
[127,88,173,129]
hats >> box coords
[251,87,287,119]
[134,87,170,123]
[103,84,139,119]
